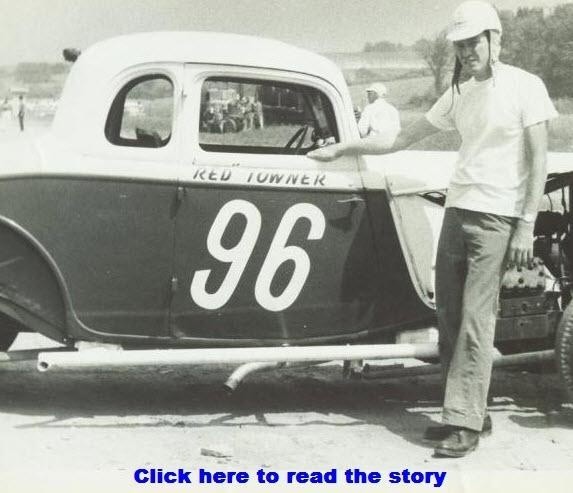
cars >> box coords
[0,29,572,402]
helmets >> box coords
[446,1,502,40]
[366,82,387,98]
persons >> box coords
[244,97,253,130]
[254,101,263,129]
[357,83,399,138]
[307,1,559,459]
[17,96,25,130]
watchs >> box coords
[520,215,536,224]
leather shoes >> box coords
[423,414,492,441]
[435,427,478,456]
